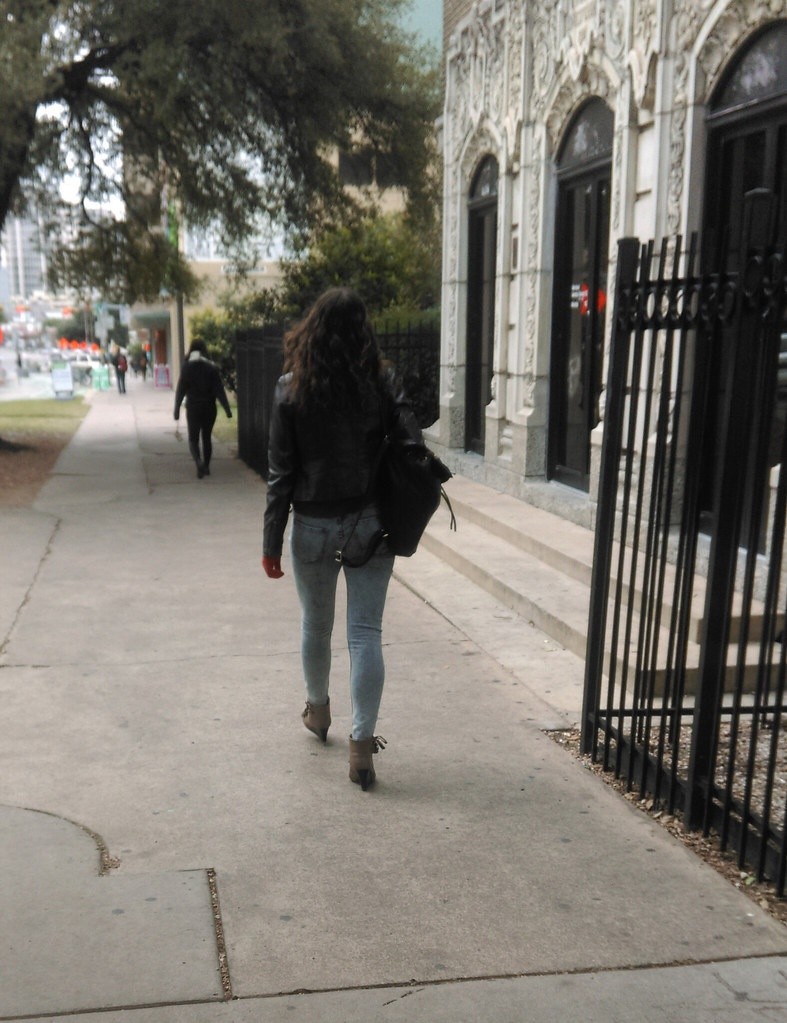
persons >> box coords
[173,338,233,479]
[261,287,428,791]
[110,346,127,393]
[130,350,149,382]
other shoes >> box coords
[203,463,210,475]
[197,463,205,479]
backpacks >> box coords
[117,355,127,372]
[334,359,457,569]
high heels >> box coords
[346,733,387,791]
[300,696,331,743]
